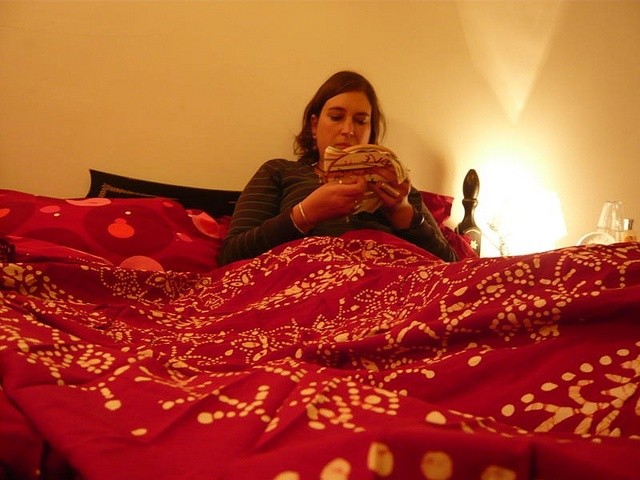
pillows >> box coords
[85,168,238,216]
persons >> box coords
[221,72,458,266]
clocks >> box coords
[577,231,617,247]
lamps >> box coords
[598,201,626,242]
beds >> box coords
[0,166,640,480]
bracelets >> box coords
[297,200,311,226]
[419,215,425,229]
